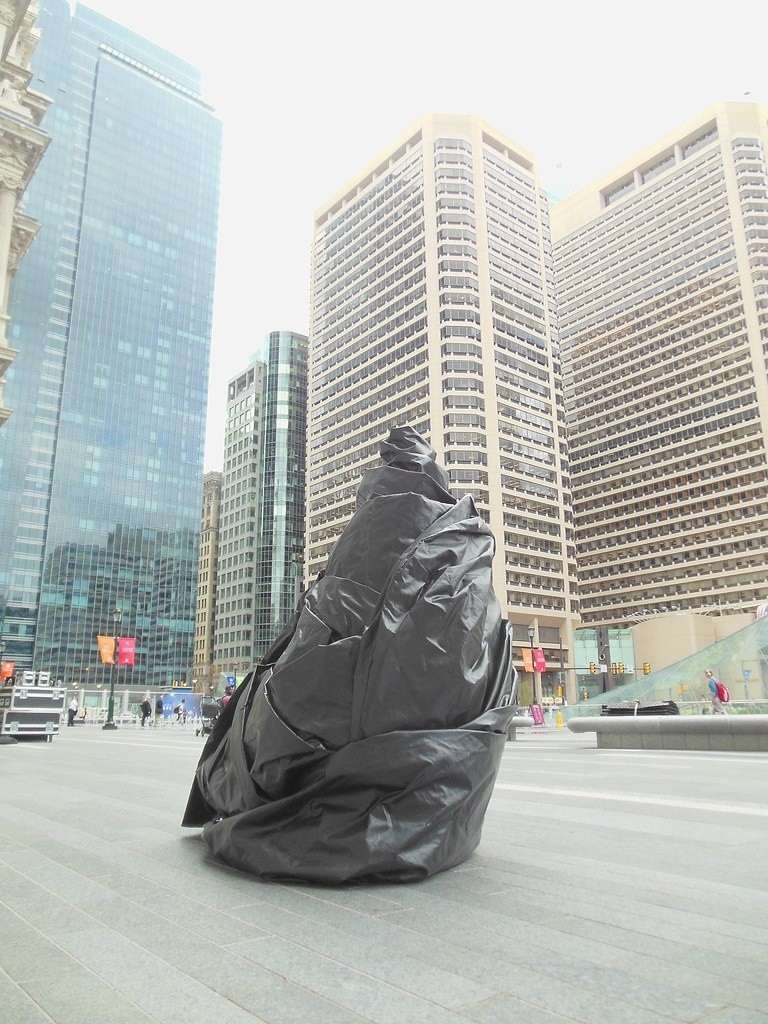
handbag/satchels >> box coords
[156,707,163,714]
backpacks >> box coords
[712,679,729,702]
[217,695,226,714]
[174,704,182,714]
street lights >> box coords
[82,668,89,708]
[72,682,77,700]
[102,607,121,730]
[527,624,538,705]
[0,641,5,669]
[192,678,197,694]
[233,667,237,691]
[96,682,100,724]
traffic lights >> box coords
[645,664,650,675]
[591,663,595,673]
[619,663,624,674]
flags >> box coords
[756,605,768,618]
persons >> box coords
[703,669,728,715]
[66,698,79,726]
[141,697,152,727]
[176,699,186,721]
[223,684,235,707]
[153,696,164,727]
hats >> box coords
[225,684,234,690]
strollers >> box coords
[195,696,220,737]
